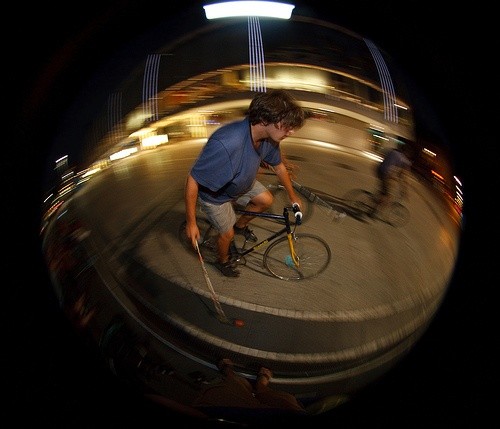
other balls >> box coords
[234,319,244,327]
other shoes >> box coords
[218,358,233,378]
[253,367,273,393]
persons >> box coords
[185,349,304,429]
[368,142,409,226]
[184,90,306,278]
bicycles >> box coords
[342,170,413,228]
[179,202,333,282]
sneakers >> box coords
[213,259,237,277]
[233,224,257,242]
